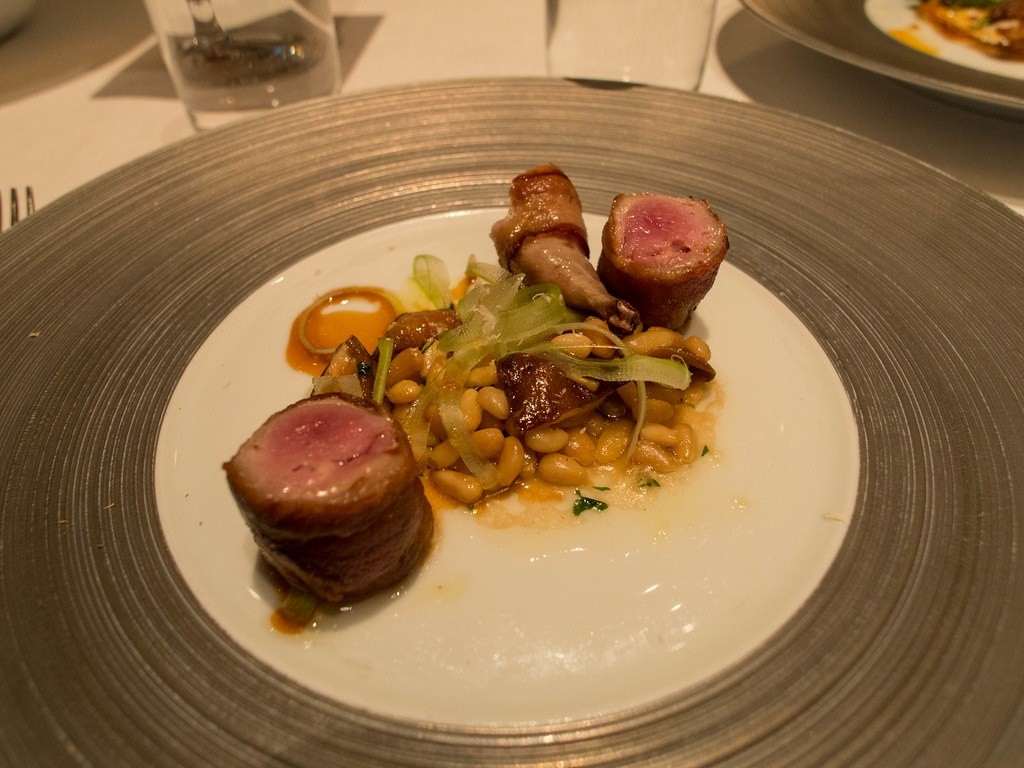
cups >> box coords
[0,76,1024,768]
[549,0,718,91]
[145,0,338,129]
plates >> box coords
[739,0,1024,117]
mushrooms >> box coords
[309,334,374,402]
[499,351,620,432]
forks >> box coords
[0,186,34,234]
